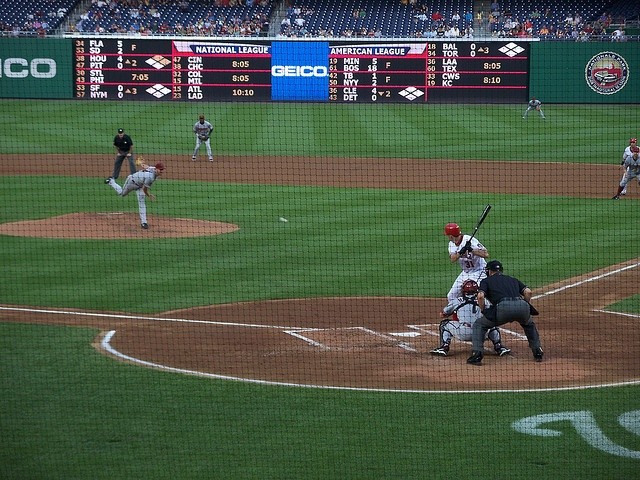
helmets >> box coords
[444,223,461,237]
[461,280,478,293]
[633,147,639,152]
[630,138,637,142]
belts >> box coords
[500,298,522,301]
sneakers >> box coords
[192,158,196,161]
[497,347,511,356]
[105,176,112,183]
[141,223,148,228]
[430,347,449,356]
[468,351,482,365]
[612,196,619,200]
[534,351,543,361]
[209,159,213,162]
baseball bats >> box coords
[464,205,492,248]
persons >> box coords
[410,4,481,38]
[467,260,544,366]
[192,115,214,162]
[276,4,308,38]
[488,1,626,39]
[178,2,190,12]
[620,139,640,194]
[612,148,640,200]
[309,26,380,38]
[444,223,492,308]
[522,97,546,119]
[216,1,267,7]
[354,8,366,18]
[112,128,137,178]
[430,279,511,356]
[68,2,172,33]
[1,5,66,35]
[175,12,269,35]
[105,154,166,229]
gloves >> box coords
[460,247,466,255]
[466,241,473,252]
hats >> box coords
[156,163,165,169]
[118,128,124,132]
[487,261,503,270]
[200,115,204,119]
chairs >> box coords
[74,1,274,37]
[280,1,473,38]
[1,0,71,37]
[489,0,609,41]
